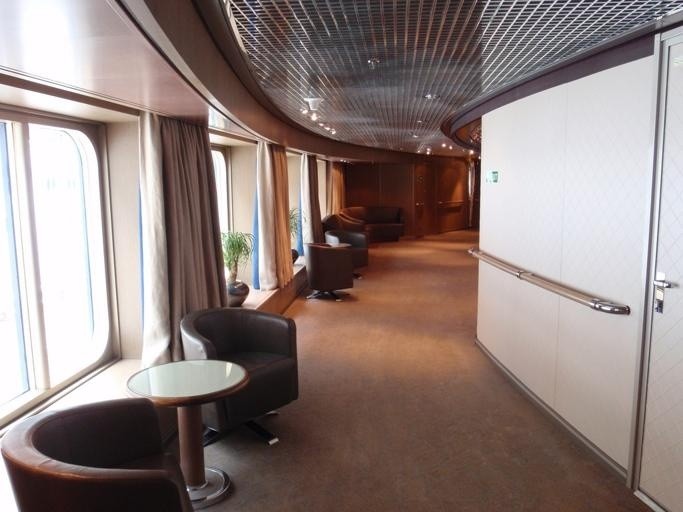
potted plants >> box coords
[289,207,306,264]
[221,231,256,306]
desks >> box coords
[125,359,249,511]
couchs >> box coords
[180,306,298,447]
[303,242,354,301]
[324,229,368,280]
[323,214,364,235]
[341,206,403,241]
[0,397,193,512]
